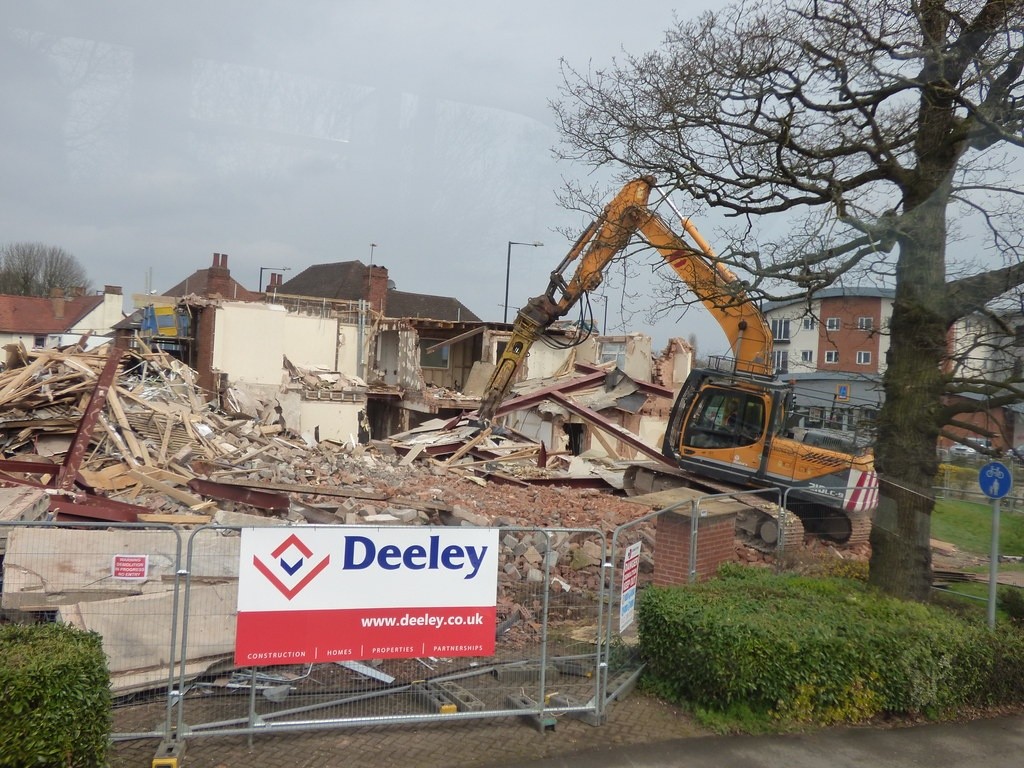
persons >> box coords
[707,401,738,431]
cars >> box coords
[1003,445,1024,464]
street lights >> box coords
[368,243,377,287]
[503,240,543,324]
[258,268,291,293]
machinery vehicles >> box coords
[469,174,879,558]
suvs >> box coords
[949,438,993,461]
